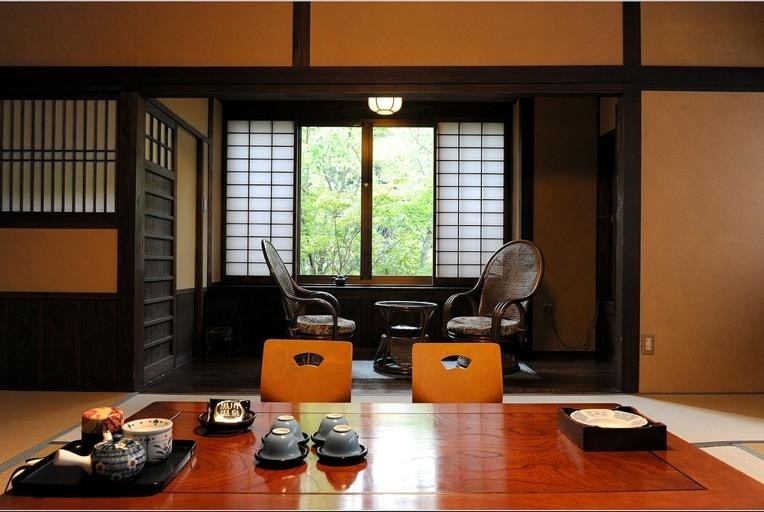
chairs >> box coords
[259,239,366,404]
[410,239,546,404]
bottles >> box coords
[92,433,145,482]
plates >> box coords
[253,432,367,464]
[568,408,647,428]
[199,410,255,433]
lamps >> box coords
[367,96,403,117]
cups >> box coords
[314,415,348,438]
[257,427,300,459]
[269,415,305,442]
[319,424,361,457]
[122,418,172,462]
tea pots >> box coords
[330,274,348,286]
